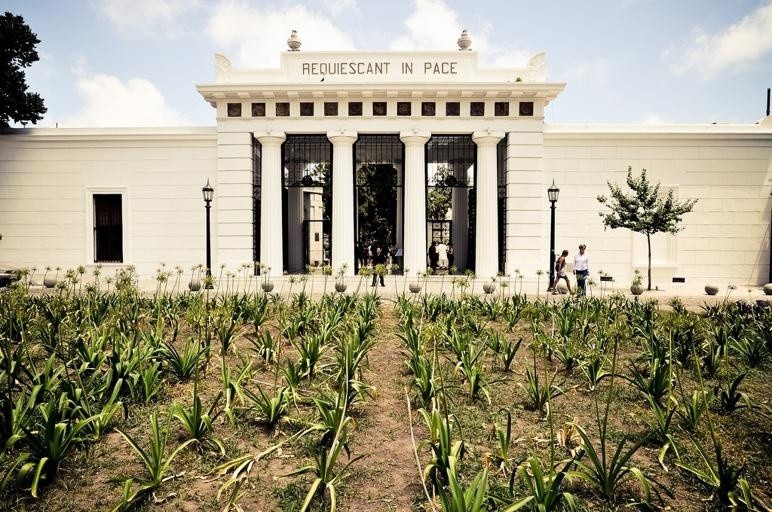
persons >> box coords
[429,241,438,275]
[573,243,591,297]
[447,242,454,273]
[360,238,398,287]
[552,250,574,296]
[435,241,449,267]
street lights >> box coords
[201,177,216,290]
[546,177,559,292]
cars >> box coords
[0,270,23,288]
